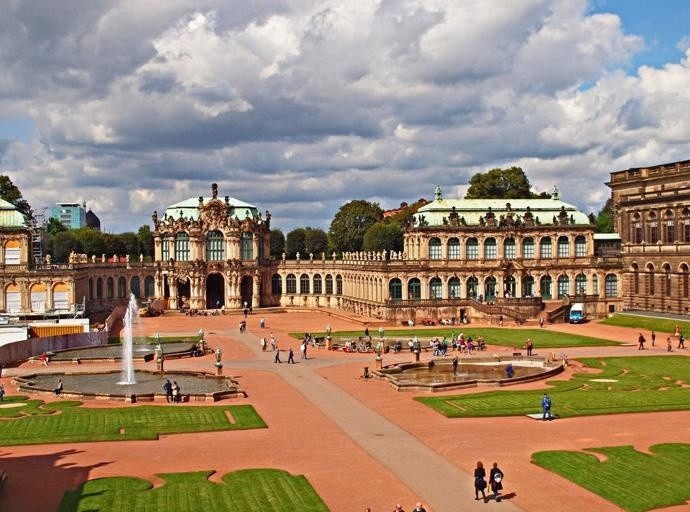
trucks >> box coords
[569,303,587,324]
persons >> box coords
[541,392,553,422]
[677,334,684,349]
[498,316,504,328]
[488,462,504,503]
[651,329,656,346]
[190,342,197,357]
[525,338,533,356]
[171,381,181,404]
[433,184,443,201]
[392,504,406,512]
[666,338,672,352]
[506,362,514,378]
[163,379,173,403]
[472,461,487,504]
[196,343,202,356]
[537,315,544,328]
[239,316,319,364]
[638,333,646,350]
[452,355,460,377]
[427,360,435,371]
[674,324,680,344]
[550,185,559,200]
[41,352,47,367]
[54,379,64,396]
[411,502,426,511]
[364,507,372,512]
[183,298,253,319]
[0,385,4,402]
[344,315,486,358]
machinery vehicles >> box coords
[139,302,160,317]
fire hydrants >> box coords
[676,325,679,333]
